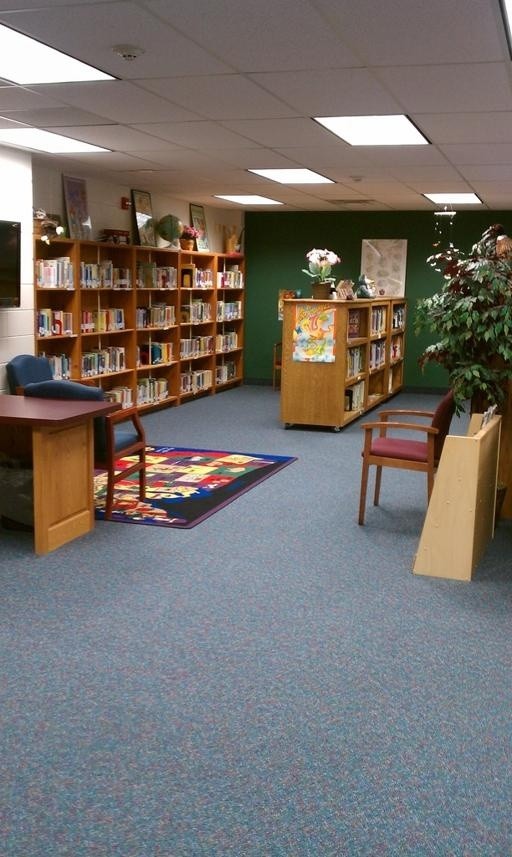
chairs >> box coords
[7,353,146,519]
[357,380,464,526]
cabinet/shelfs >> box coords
[280,296,409,431]
[32,233,246,424]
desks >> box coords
[0,394,122,555]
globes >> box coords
[159,214,184,249]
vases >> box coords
[180,236,196,251]
[310,279,332,300]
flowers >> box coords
[181,224,201,240]
[301,247,340,281]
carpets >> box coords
[80,444,300,530]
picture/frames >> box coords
[130,188,157,247]
[61,175,94,240]
[359,237,409,300]
[190,202,211,252]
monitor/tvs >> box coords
[0,221,20,308]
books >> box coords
[35,257,244,409]
[344,307,406,411]
